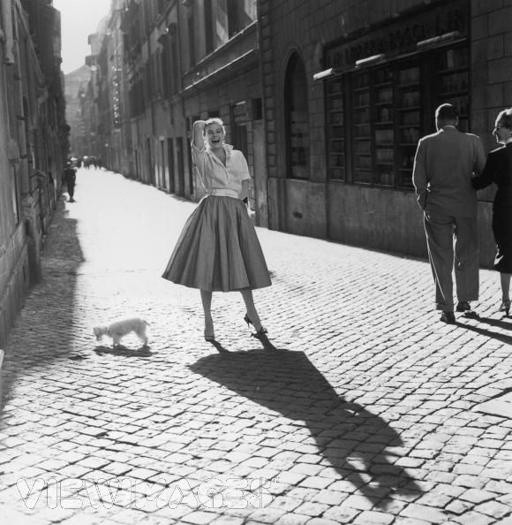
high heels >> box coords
[205,330,214,341]
[244,313,268,334]
[499,303,510,316]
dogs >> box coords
[92,317,152,347]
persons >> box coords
[474,106,512,318]
[63,161,76,204]
[159,116,274,345]
[410,101,486,325]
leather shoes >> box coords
[440,311,455,324]
[456,301,470,312]
